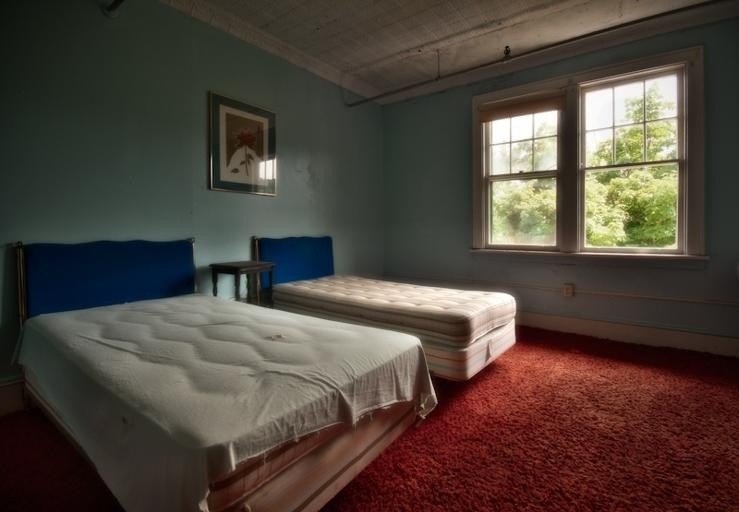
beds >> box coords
[250,235,517,385]
[13,239,428,512]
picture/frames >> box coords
[208,87,277,197]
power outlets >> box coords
[562,284,575,297]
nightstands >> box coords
[209,260,274,306]
